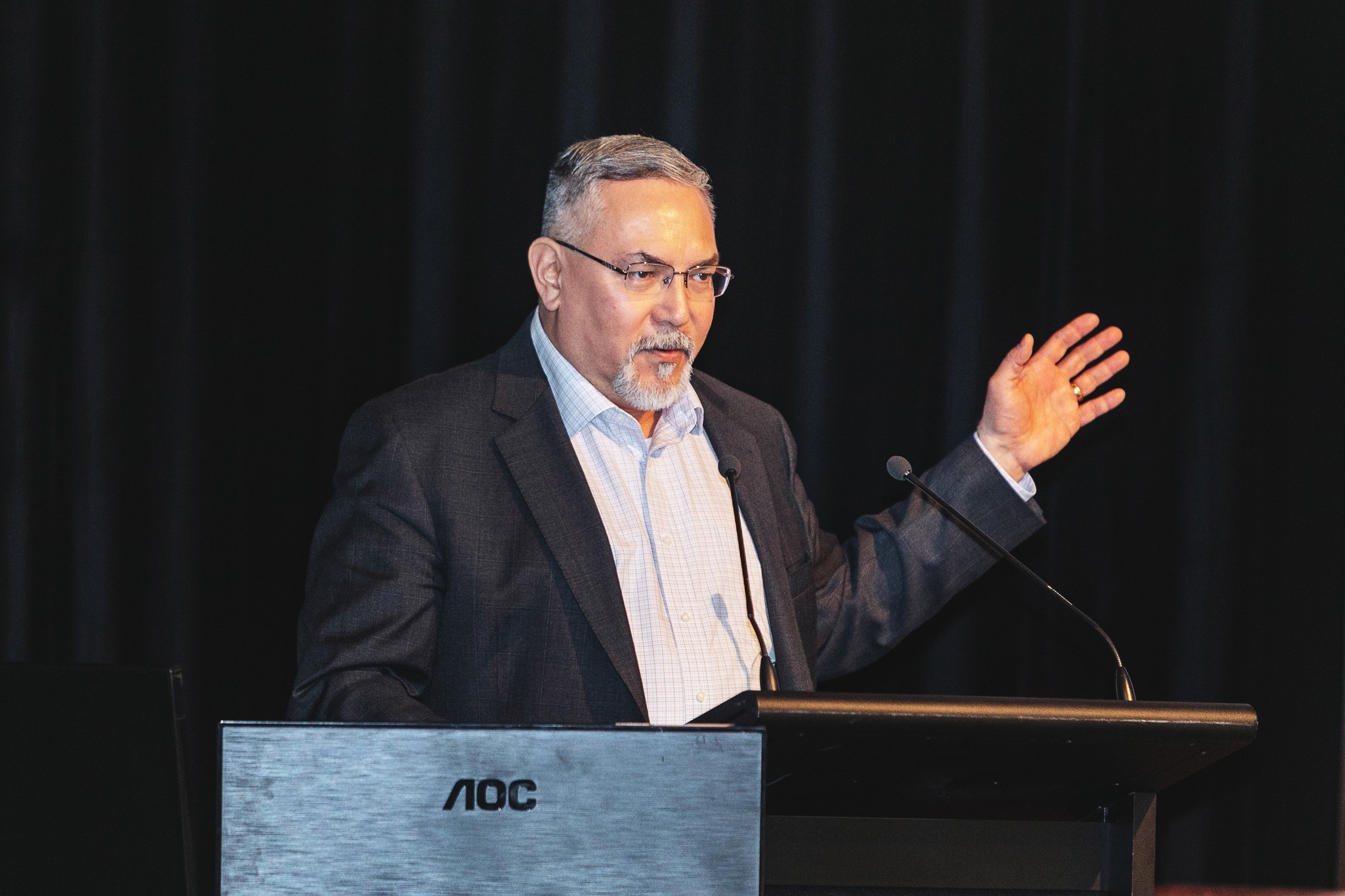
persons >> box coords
[280,133,1130,731]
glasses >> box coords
[556,239,734,302]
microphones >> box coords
[886,456,1136,699]
[717,455,781,689]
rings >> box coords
[1069,380,1084,404]
